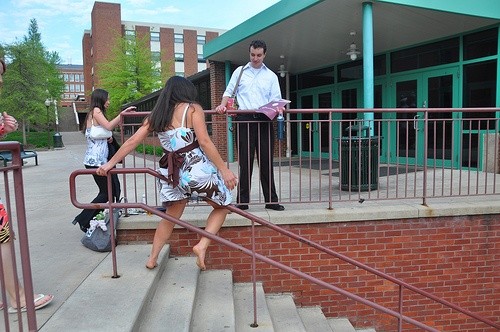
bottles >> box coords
[277,114,284,141]
[141,194,145,204]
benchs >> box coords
[0,141,37,167]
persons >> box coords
[96,76,238,270]
[72,88,136,232]
[216,41,285,210]
[0,59,54,312]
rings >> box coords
[15,120,17,123]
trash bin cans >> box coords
[331,124,383,192]
[52,132,63,148]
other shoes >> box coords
[265,204,284,210]
[230,205,249,211]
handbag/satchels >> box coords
[227,98,238,117]
[80,212,119,252]
[90,113,113,139]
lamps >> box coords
[278,56,288,77]
[346,32,360,60]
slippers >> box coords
[8,293,55,313]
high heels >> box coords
[72,218,90,233]
